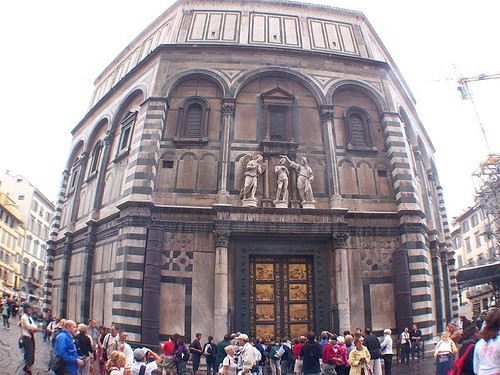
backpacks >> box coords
[448,343,474,375]
[182,351,189,362]
[203,344,213,357]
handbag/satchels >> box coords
[48,356,67,375]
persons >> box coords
[235,334,254,375]
[271,158,290,202]
[131,347,161,375]
[474,306,500,375]
[0,291,487,375]
[279,153,315,203]
[55,320,85,375]
[242,153,263,199]
[19,305,42,375]
